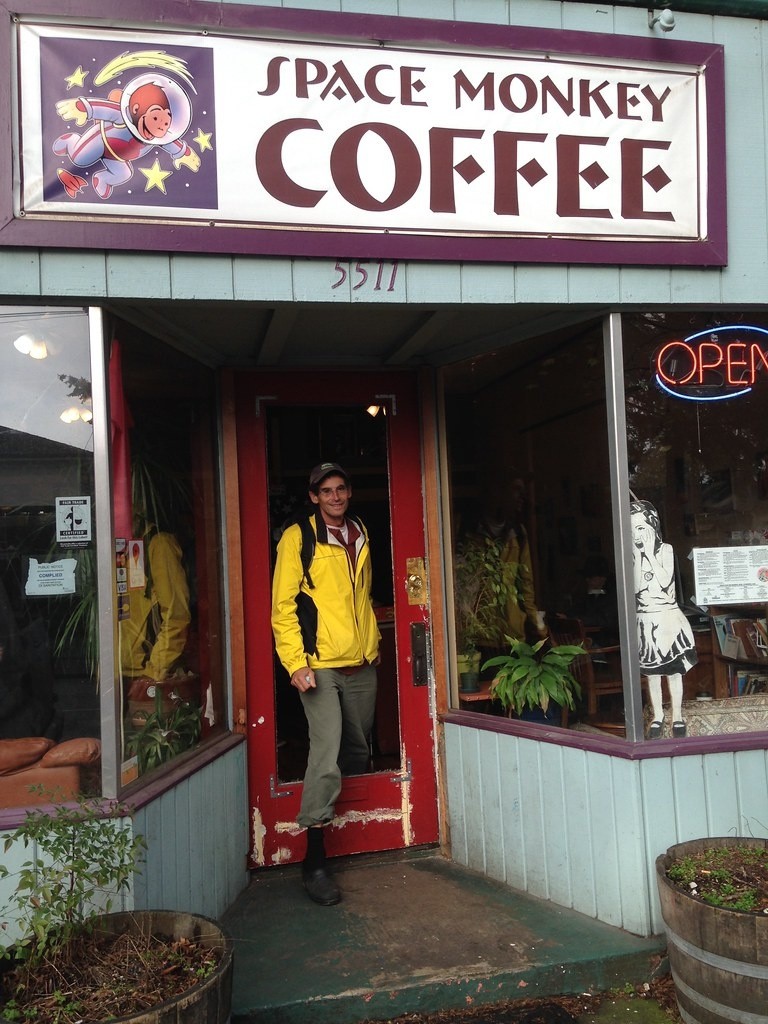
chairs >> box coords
[542,617,625,729]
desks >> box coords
[584,708,626,739]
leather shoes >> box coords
[300,860,341,906]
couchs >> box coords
[0,762,81,810]
[569,692,768,740]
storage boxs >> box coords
[692,544,768,606]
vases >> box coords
[657,835,768,1024]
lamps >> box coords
[59,404,94,425]
[13,312,75,360]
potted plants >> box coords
[452,515,531,693]
[483,633,590,728]
[0,783,234,1024]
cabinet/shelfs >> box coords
[709,604,768,700]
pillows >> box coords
[0,737,56,776]
[40,738,101,768]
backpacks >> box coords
[270,514,366,590]
[144,524,198,632]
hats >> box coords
[309,462,351,487]
[507,467,537,483]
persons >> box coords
[113,513,196,798]
[272,462,381,907]
